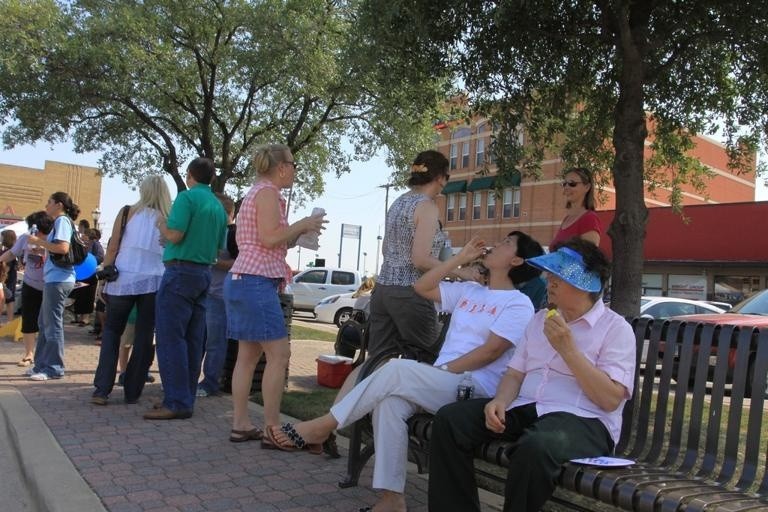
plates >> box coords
[573,455,635,470]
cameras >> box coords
[94,266,119,283]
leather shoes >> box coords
[88,397,107,405]
[79,320,90,327]
[143,407,193,420]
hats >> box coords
[525,246,608,294]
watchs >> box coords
[440,364,448,373]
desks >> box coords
[17,270,25,281]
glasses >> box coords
[281,160,297,169]
[443,172,450,181]
[561,179,585,188]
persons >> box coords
[26,192,76,380]
[323,149,451,459]
[0,229,18,322]
[0,283,5,313]
[266,231,546,510]
[427,237,638,512]
[196,192,240,399]
[0,211,54,367]
[91,173,165,404]
[116,303,156,386]
[350,276,375,348]
[142,157,229,418]
[222,143,330,450]
[549,167,601,252]
[69,220,113,339]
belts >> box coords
[162,259,206,266]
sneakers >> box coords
[88,329,96,336]
[25,368,35,375]
[31,371,65,381]
[195,382,209,397]
[323,432,343,459]
[145,372,155,382]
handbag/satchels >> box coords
[339,311,367,349]
[49,214,87,268]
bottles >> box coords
[457,370,475,402]
[28,224,39,249]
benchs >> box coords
[338,312,768,511]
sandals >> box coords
[261,434,278,451]
[229,422,264,442]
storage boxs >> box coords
[316,354,355,388]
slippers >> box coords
[94,334,102,339]
[17,359,33,367]
[266,422,323,455]
[359,504,409,512]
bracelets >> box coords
[157,222,161,226]
